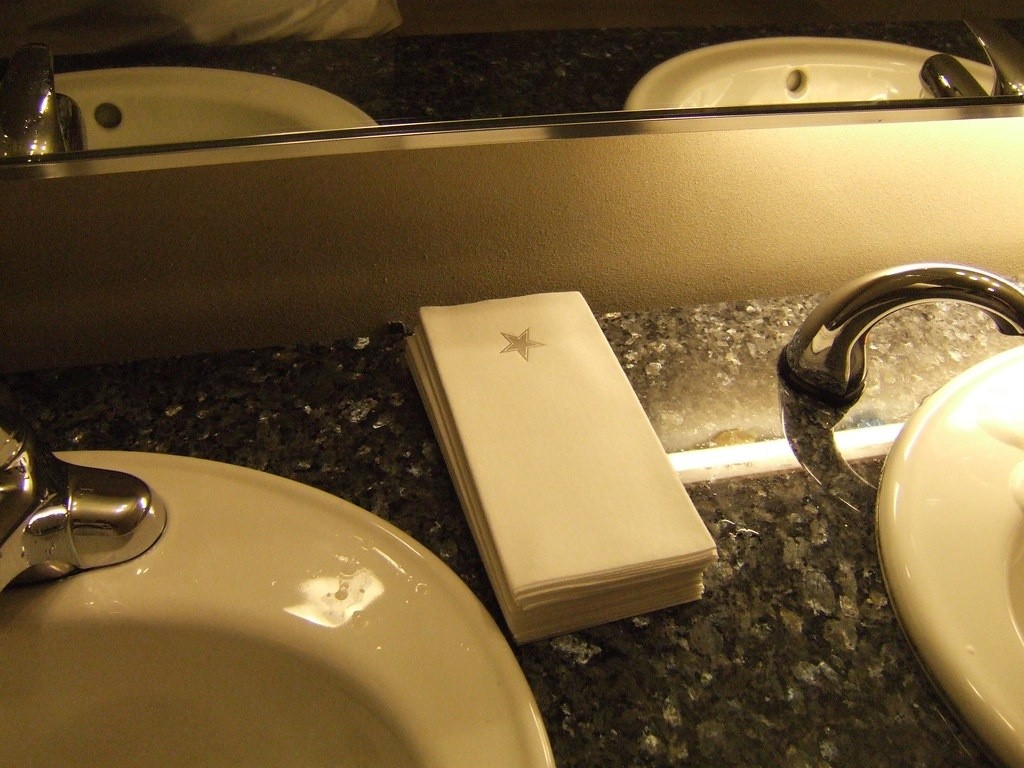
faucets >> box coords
[919,10,1024,99]
[0,420,167,600]
[774,258,1024,426]
[0,43,88,156]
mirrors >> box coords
[0,0,1024,167]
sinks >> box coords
[0,449,560,768]
[871,340,1024,768]
[622,32,997,113]
[54,65,380,150]
